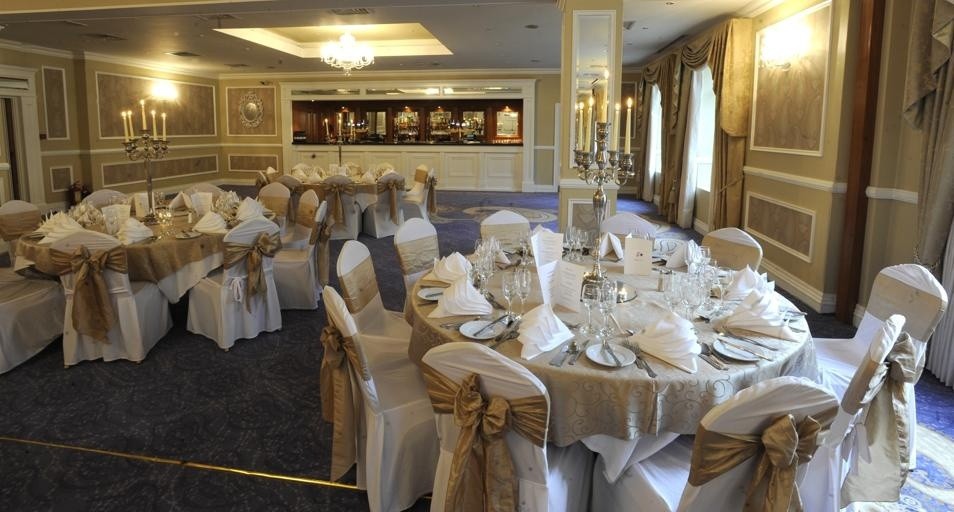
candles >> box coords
[594,68,609,123]
[350,119,353,135]
[120,98,168,142]
[575,101,584,151]
[582,96,594,153]
[325,118,330,137]
[608,103,621,151]
[338,120,342,136]
[624,97,634,154]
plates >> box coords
[587,343,636,368]
[716,335,765,363]
[418,286,447,305]
[459,318,506,341]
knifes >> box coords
[549,337,589,367]
[472,312,510,338]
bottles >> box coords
[658,267,674,294]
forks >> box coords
[712,324,778,353]
[622,338,657,380]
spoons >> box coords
[486,322,522,354]
[696,341,729,367]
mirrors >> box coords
[569,8,618,170]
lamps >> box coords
[320,31,376,78]
[761,30,803,73]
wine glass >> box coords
[282,164,386,184]
[472,232,536,320]
[79,190,226,238]
[566,226,600,263]
[580,277,621,336]
[665,246,735,323]
